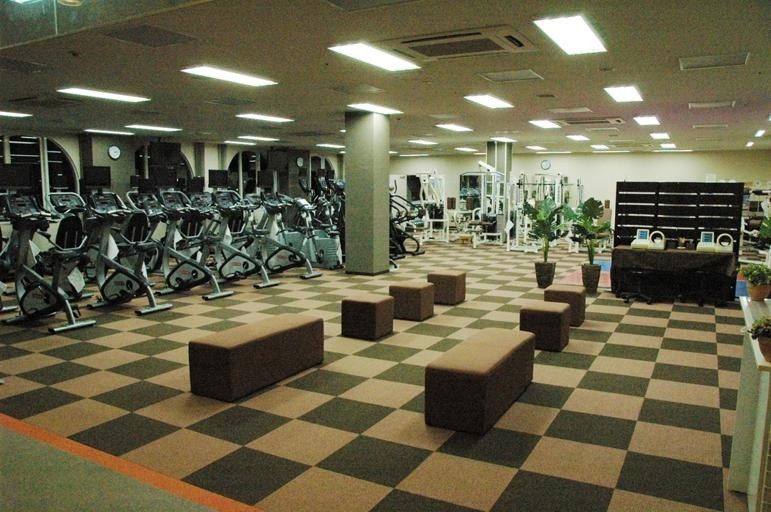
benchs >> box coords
[189,316,324,402]
[424,327,534,433]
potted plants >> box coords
[528,195,611,292]
[738,262,771,302]
[748,315,771,364]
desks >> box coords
[612,245,737,301]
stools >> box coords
[621,266,729,307]
[341,271,589,352]
